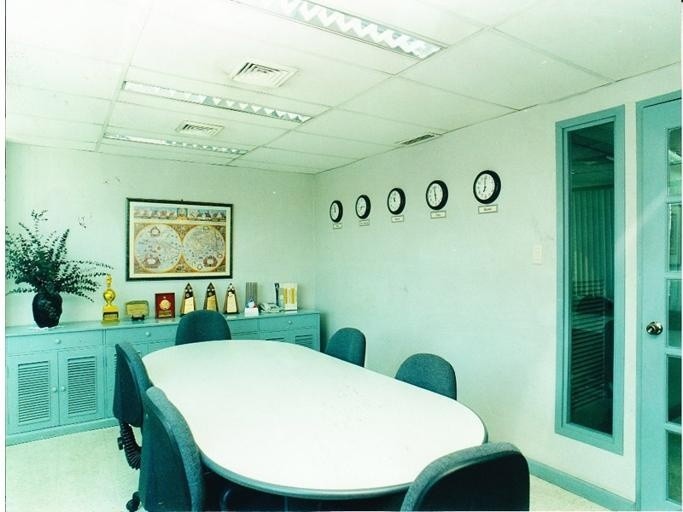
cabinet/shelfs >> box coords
[260,316,320,352]
[226,320,260,340]
[5,329,105,438]
[105,325,178,420]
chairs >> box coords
[124,383,215,511]
[173,310,231,347]
[394,352,457,401]
[111,341,155,471]
[398,442,529,511]
[324,327,366,367]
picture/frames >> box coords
[124,197,233,281]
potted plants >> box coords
[4,206,115,330]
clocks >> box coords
[328,169,501,223]
